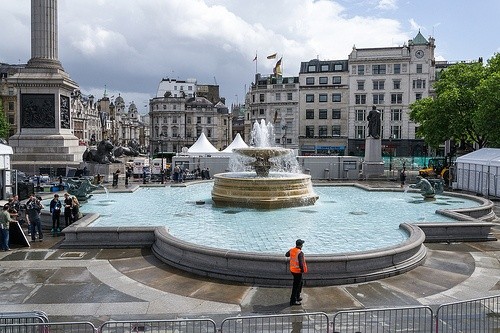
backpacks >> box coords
[50,200,56,213]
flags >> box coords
[252,55,258,61]
[266,53,277,59]
[273,60,282,76]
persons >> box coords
[423,165,427,169]
[367,105,381,139]
[410,176,435,194]
[197,164,211,179]
[285,239,307,306]
[400,169,407,188]
[403,161,406,171]
[143,171,147,184]
[125,172,128,184]
[0,192,80,251]
[164,166,190,183]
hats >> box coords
[296,239,304,246]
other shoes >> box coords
[31,240,35,242]
[37,232,39,235]
[290,301,301,305]
[57,228,60,231]
[2,249,5,251]
[28,233,31,235]
[51,229,55,232]
[296,297,303,301]
[39,239,43,242]
[6,249,13,250]
